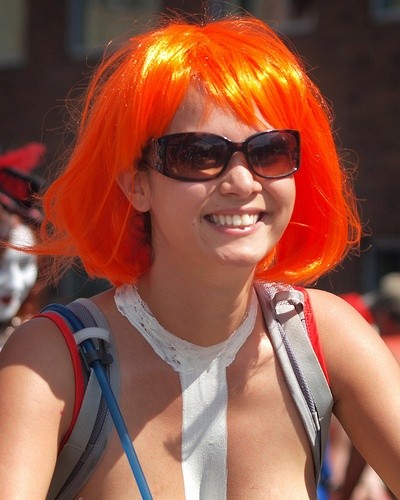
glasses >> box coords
[135,130,303,182]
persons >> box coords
[1,17,399,500]
[0,142,43,344]
[333,270,399,500]
